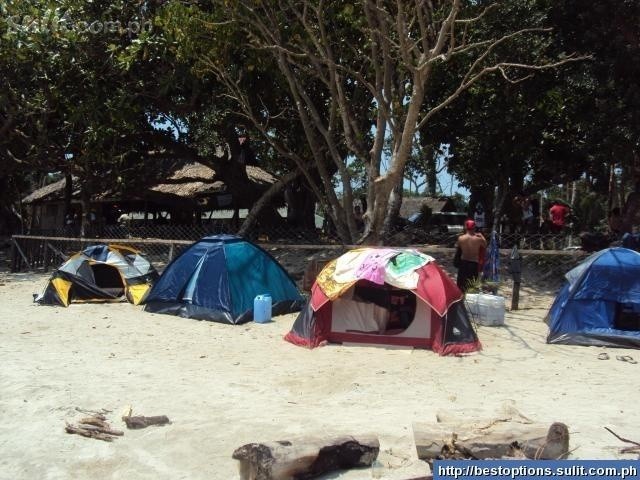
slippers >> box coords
[616,355,637,364]
[598,352,610,360]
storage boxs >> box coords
[464,293,504,326]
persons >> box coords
[455,189,640,291]
[353,205,364,227]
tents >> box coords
[284,245,483,356]
[543,246,640,350]
[33,244,160,307]
[142,234,307,325]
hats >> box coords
[465,220,476,229]
